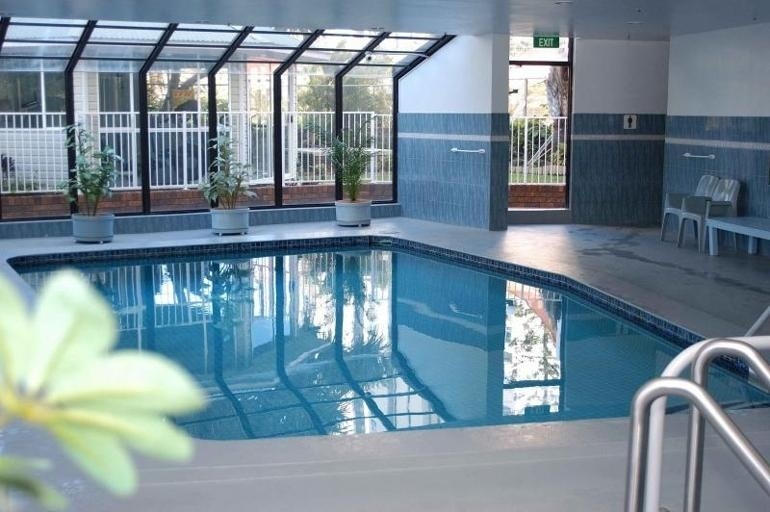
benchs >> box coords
[705,216,770,256]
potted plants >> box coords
[304,115,384,227]
[56,122,124,244]
[204,251,371,343]
[197,125,259,236]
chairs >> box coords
[660,174,720,240]
[677,179,740,251]
